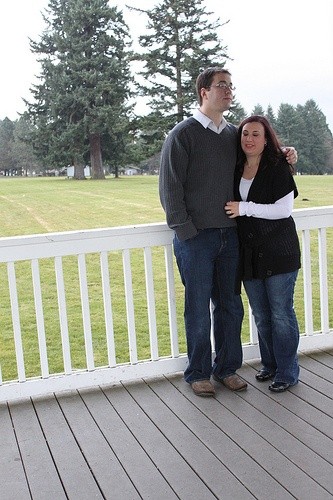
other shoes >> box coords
[191,379,215,399]
[218,374,249,393]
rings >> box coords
[230,211,232,214]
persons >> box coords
[225,116,301,393]
[158,66,298,397]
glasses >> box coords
[204,81,237,92]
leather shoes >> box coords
[255,368,274,381]
[268,380,292,393]
